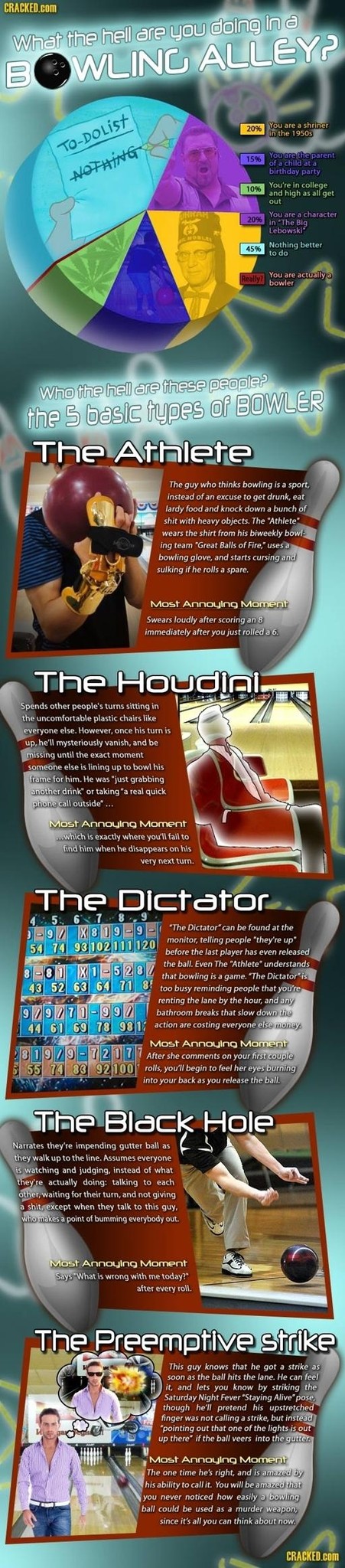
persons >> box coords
[19,462,147,649]
[67,1359,123,1424]
[124,231,169,318]
[152,127,255,213]
[181,1128,309,1279]
[17,1410,89,1539]
[166,207,238,320]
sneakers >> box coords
[203,1214,225,1237]
[221,1250,253,1279]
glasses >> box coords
[87,1371,102,1377]
[188,146,218,161]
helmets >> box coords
[43,463,135,550]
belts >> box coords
[30,1500,70,1508]
